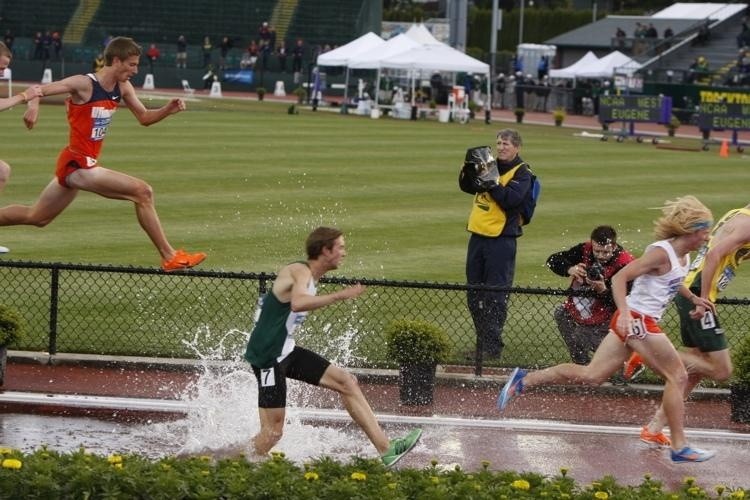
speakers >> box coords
[497,9,502,30]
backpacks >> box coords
[513,161,542,226]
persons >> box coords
[458,128,539,362]
[0,34,209,275]
[621,202,750,450]
[496,193,717,465]
[223,228,424,468]
[546,223,647,383]
[0,41,45,254]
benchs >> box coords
[651,14,750,93]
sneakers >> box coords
[497,365,528,411]
[639,425,671,448]
[669,443,717,463]
[0,245,11,252]
[381,429,422,468]
[161,249,210,272]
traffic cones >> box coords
[719,138,732,157]
[140,74,155,91]
[39,68,53,85]
[208,81,223,98]
[273,80,287,98]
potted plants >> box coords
[256,86,266,100]
[550,104,566,126]
[666,114,679,136]
[294,87,307,104]
[514,110,524,121]
[385,319,456,405]
[728,337,750,424]
[469,99,481,119]
[0,301,25,391]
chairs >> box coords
[3,2,366,84]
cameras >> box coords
[583,266,606,286]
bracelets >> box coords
[17,91,28,104]
[687,294,697,302]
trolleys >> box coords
[597,93,673,145]
